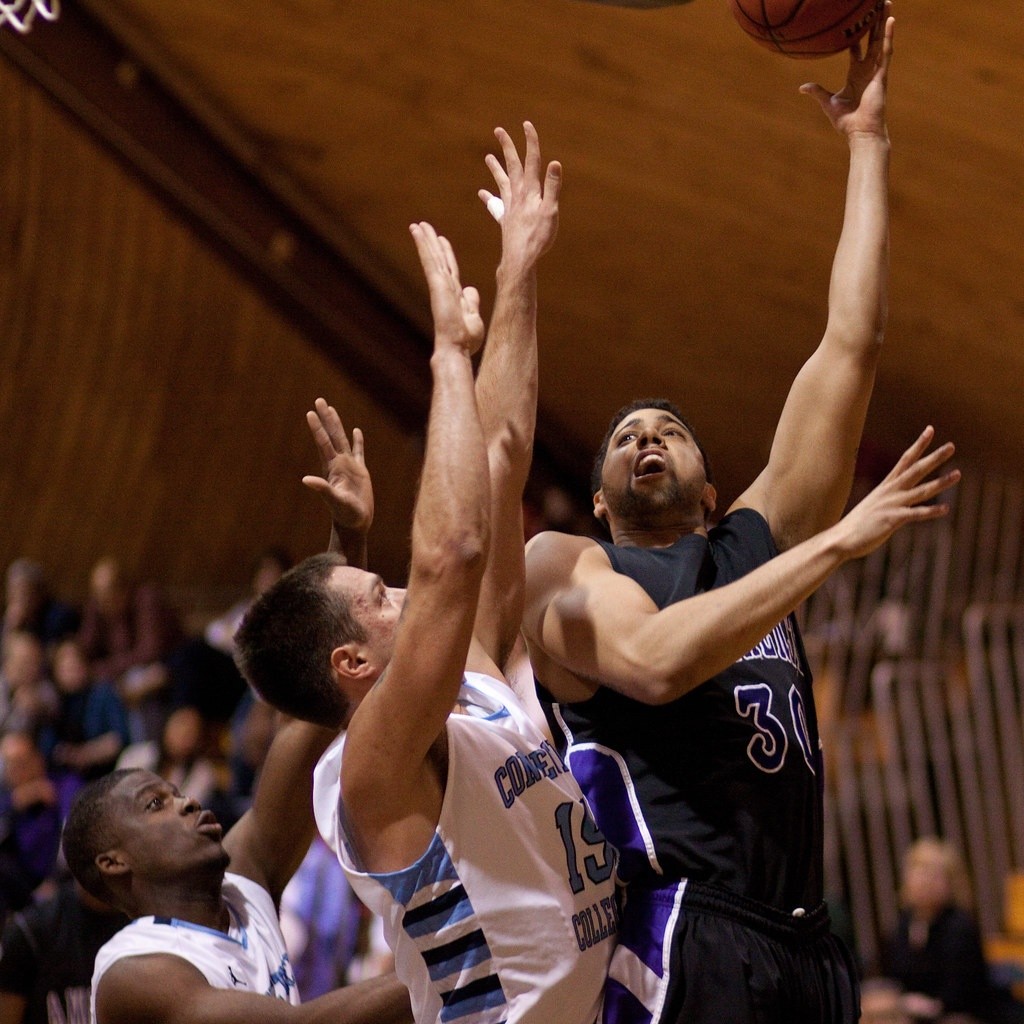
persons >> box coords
[233,120,621,1023]
[61,397,411,1023]
[519,1,963,1024]
[1,544,299,1023]
[878,835,998,1024]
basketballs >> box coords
[731,0,885,61]
